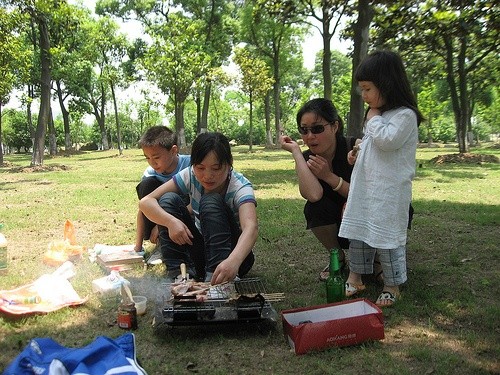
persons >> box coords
[133,125,195,266]
[281,98,414,286]
[337,50,427,309]
[138,132,258,286]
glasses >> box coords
[298,123,331,135]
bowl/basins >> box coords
[121,296,147,315]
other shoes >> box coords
[147,250,165,265]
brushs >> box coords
[122,283,137,305]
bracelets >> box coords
[333,177,343,191]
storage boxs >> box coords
[281,297,385,356]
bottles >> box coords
[0,225,7,271]
[327,248,344,303]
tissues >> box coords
[91,269,130,299]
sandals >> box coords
[344,282,366,296]
[319,253,348,281]
[375,287,402,307]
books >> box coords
[96,244,144,274]
